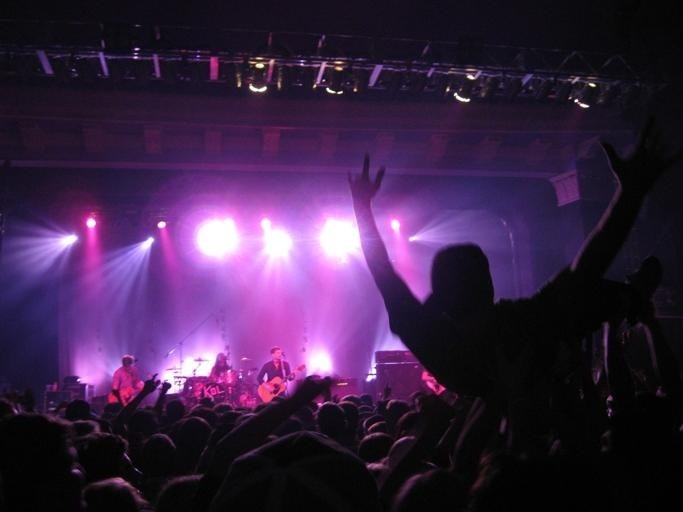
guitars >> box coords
[258,365,305,403]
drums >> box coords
[183,377,207,400]
[219,367,257,386]
[200,383,225,408]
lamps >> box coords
[450,70,478,105]
[245,58,271,95]
[572,77,595,110]
[323,64,348,95]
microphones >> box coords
[281,351,287,356]
